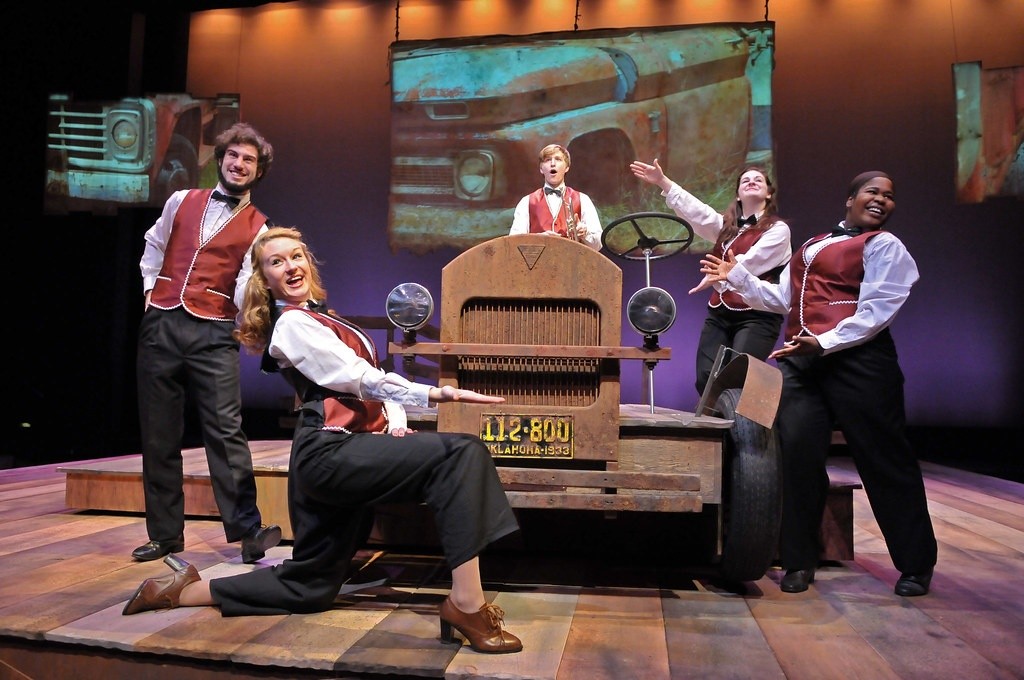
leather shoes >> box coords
[130,531,185,561]
[895,566,934,596]
[778,567,815,592]
[242,523,282,563]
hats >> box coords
[844,155,888,182]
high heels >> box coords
[440,594,524,655]
[123,551,201,615]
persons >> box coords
[121,225,524,655]
[509,143,604,254]
[129,123,278,565]
[629,157,794,415]
[699,169,939,598]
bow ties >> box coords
[545,187,562,196]
[831,225,863,237]
[210,190,241,210]
[737,213,758,227]
[307,296,327,314]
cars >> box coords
[376,212,785,582]
[394,25,752,212]
[42,91,240,211]
